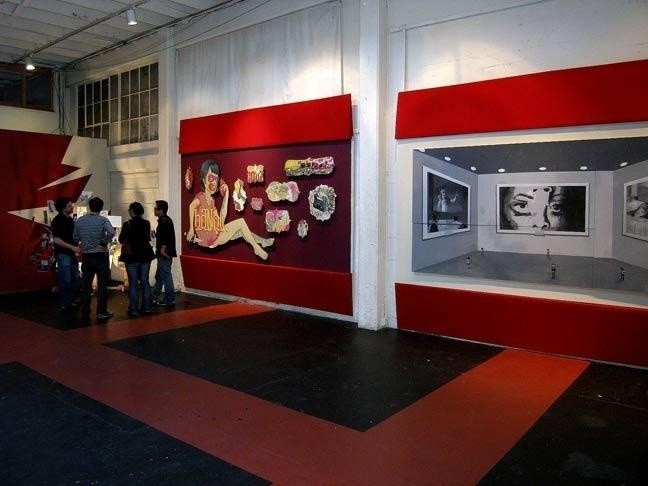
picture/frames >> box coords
[495,182,589,237]
[621,176,647,243]
[421,164,470,240]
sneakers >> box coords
[127,299,175,318]
[96,312,113,319]
[59,301,91,314]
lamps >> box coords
[12,0,149,70]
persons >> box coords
[117,201,157,317]
[151,200,179,306]
[72,197,115,319]
[502,186,573,228]
[50,197,82,306]
[185,158,275,261]
[429,187,467,232]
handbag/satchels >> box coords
[120,221,134,257]
